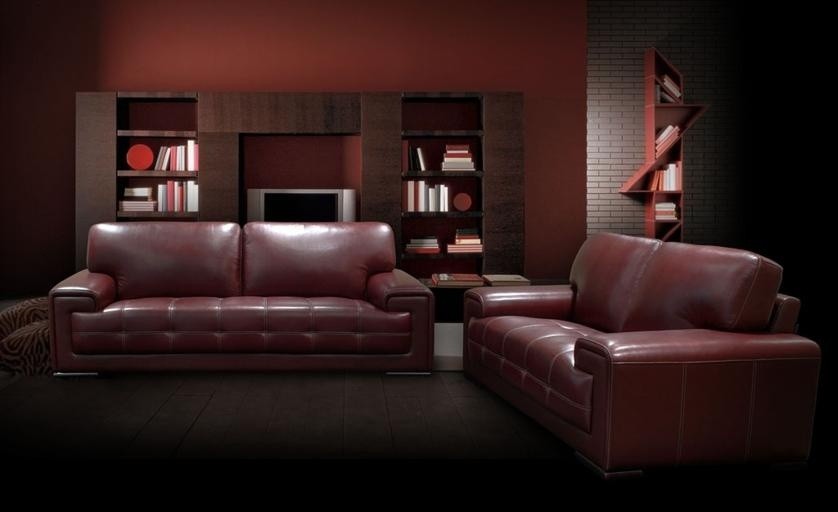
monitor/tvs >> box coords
[247,187,356,223]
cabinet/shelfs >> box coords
[401,90,484,289]
[620,48,711,243]
[116,91,199,219]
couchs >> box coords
[463,232,822,487]
[49,221,435,378]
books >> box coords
[432,273,485,287]
[647,73,683,221]
[401,139,484,254]
[117,138,200,212]
[482,274,531,287]
